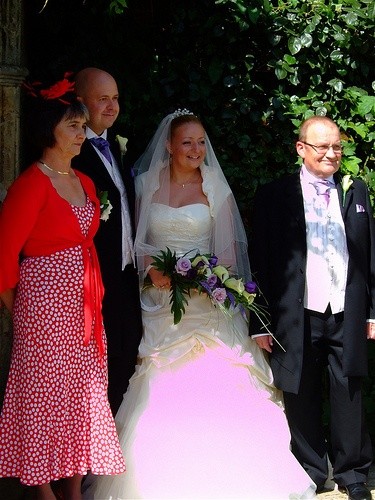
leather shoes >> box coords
[338,482,372,500]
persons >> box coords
[82,108,317,500]
[248,116,375,500]
[71,67,144,419]
[0,72,126,500]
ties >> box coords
[312,183,333,211]
[88,137,112,167]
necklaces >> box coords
[38,159,73,175]
[170,176,200,188]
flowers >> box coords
[141,247,286,351]
[341,175,353,207]
[116,133,128,158]
[97,191,113,222]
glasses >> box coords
[300,141,342,154]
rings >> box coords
[160,282,171,289]
